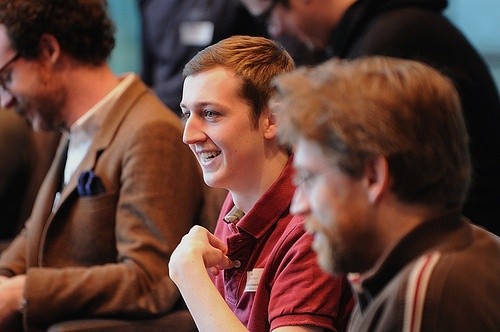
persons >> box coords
[0,0,229,332]
[168,36,357,332]
[144,0,272,121]
[268,57,500,332]
[240,0,500,237]
[0,106,63,242]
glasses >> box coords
[0,53,23,90]
[255,0,281,24]
